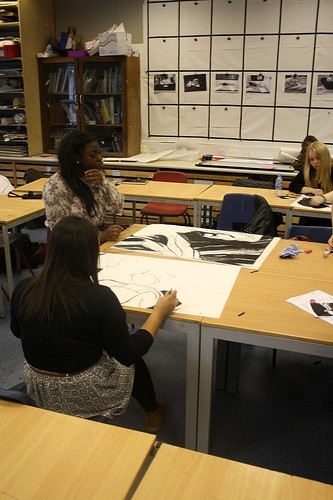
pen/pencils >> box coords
[168,288,172,294]
[113,213,116,225]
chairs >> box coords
[217,194,270,235]
[140,171,191,226]
[287,226,332,244]
[248,174,283,227]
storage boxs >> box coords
[99,33,131,57]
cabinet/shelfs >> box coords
[0,0,56,158]
[39,55,142,158]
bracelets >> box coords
[321,196,326,203]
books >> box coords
[54,127,75,150]
[85,67,122,94]
[48,68,68,93]
[84,96,120,125]
[49,99,77,123]
[110,131,122,152]
[0,145,28,158]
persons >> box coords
[10,215,177,432]
[292,135,318,171]
[42,131,125,245]
[0,176,27,233]
[23,169,43,184]
[288,141,333,227]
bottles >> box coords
[276,173,283,197]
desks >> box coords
[0,160,333,500]
